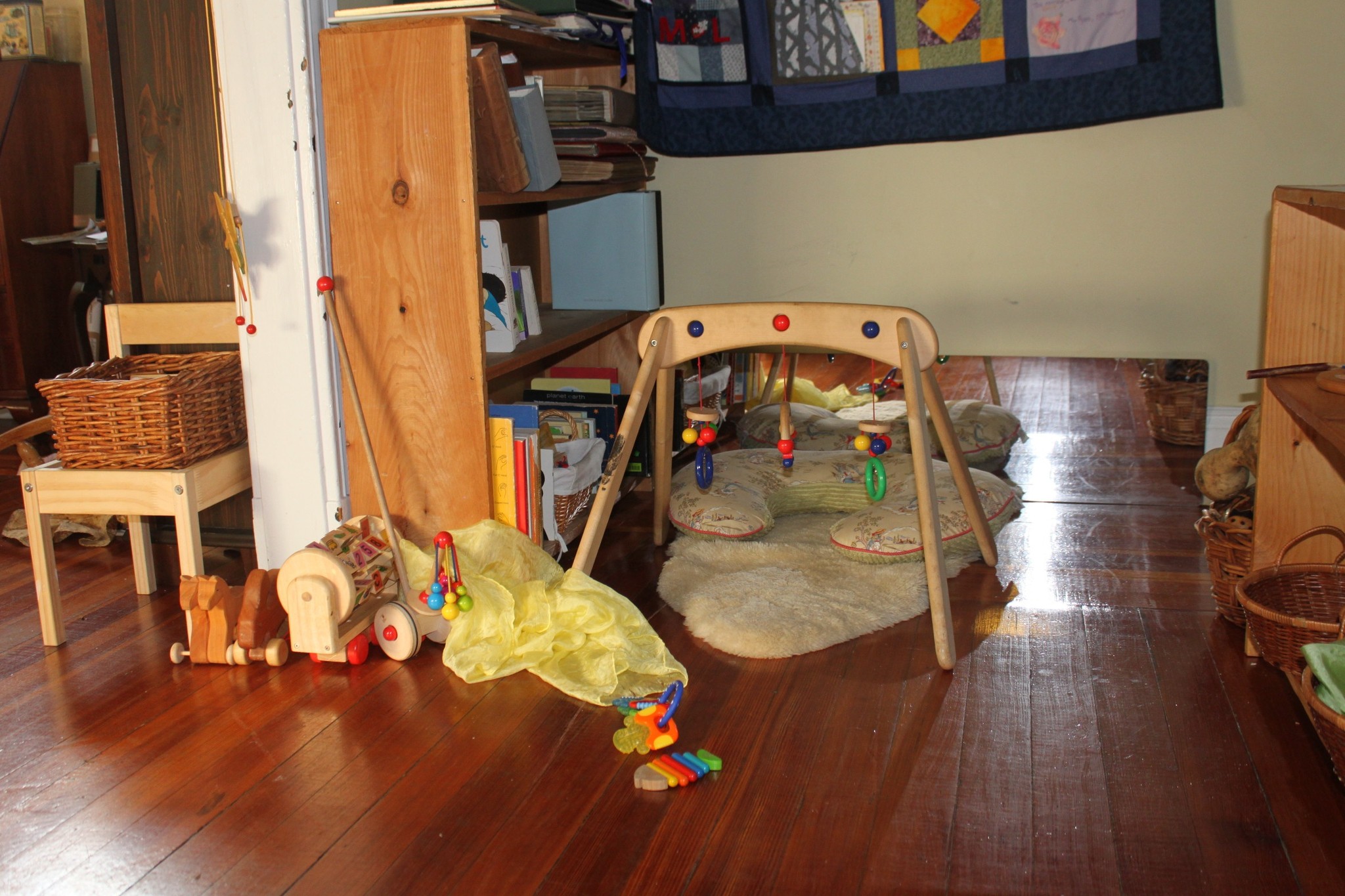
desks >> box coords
[0,55,107,475]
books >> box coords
[470,42,658,193]
[326,0,639,55]
[487,366,653,550]
[479,219,543,352]
[547,190,665,312]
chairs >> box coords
[19,298,256,649]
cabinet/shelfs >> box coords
[313,11,671,587]
[1238,178,1345,781]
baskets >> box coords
[537,408,605,535]
[1301,664,1344,784]
[1193,480,1254,629]
[1235,525,1345,677]
[1137,358,1209,446]
[34,350,247,471]
